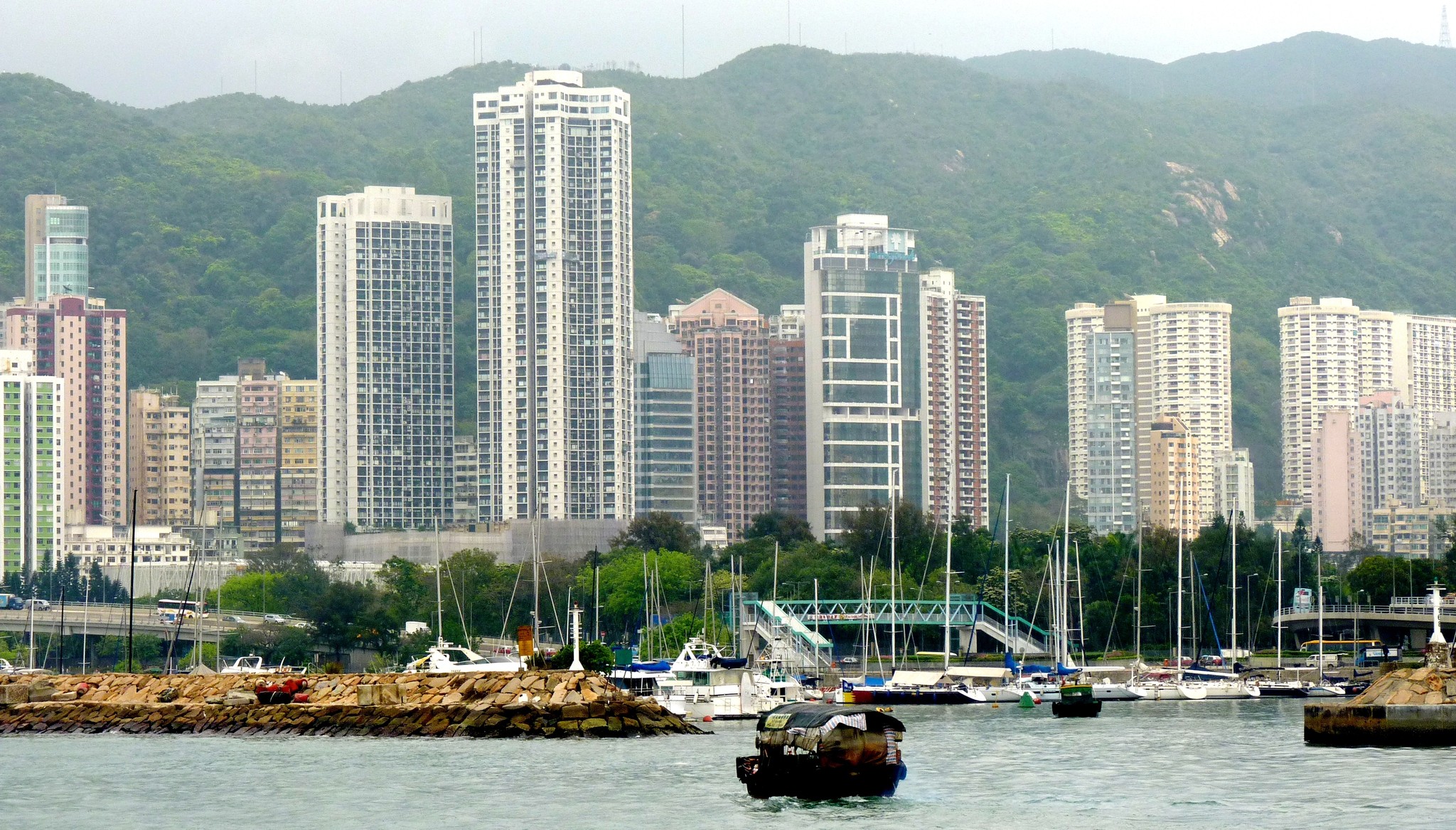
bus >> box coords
[158,599,209,620]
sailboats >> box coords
[399,516,567,673]
[176,492,307,674]
[0,596,53,675]
[600,466,1374,801]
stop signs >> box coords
[601,631,605,636]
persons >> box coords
[821,740,845,767]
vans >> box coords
[25,599,50,611]
[159,613,184,625]
[1306,653,1349,669]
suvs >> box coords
[265,614,286,625]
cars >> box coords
[222,616,245,624]
[294,622,317,630]
[493,645,511,653]
[541,648,557,656]
[1171,656,1194,666]
[1200,655,1227,666]
[838,657,860,664]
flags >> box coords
[842,679,853,693]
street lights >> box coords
[1197,573,1209,654]
[1246,573,1259,667]
[1357,590,1365,667]
[1272,549,1290,581]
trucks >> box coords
[1354,644,1402,667]
[1220,649,1254,659]
[0,594,23,611]
